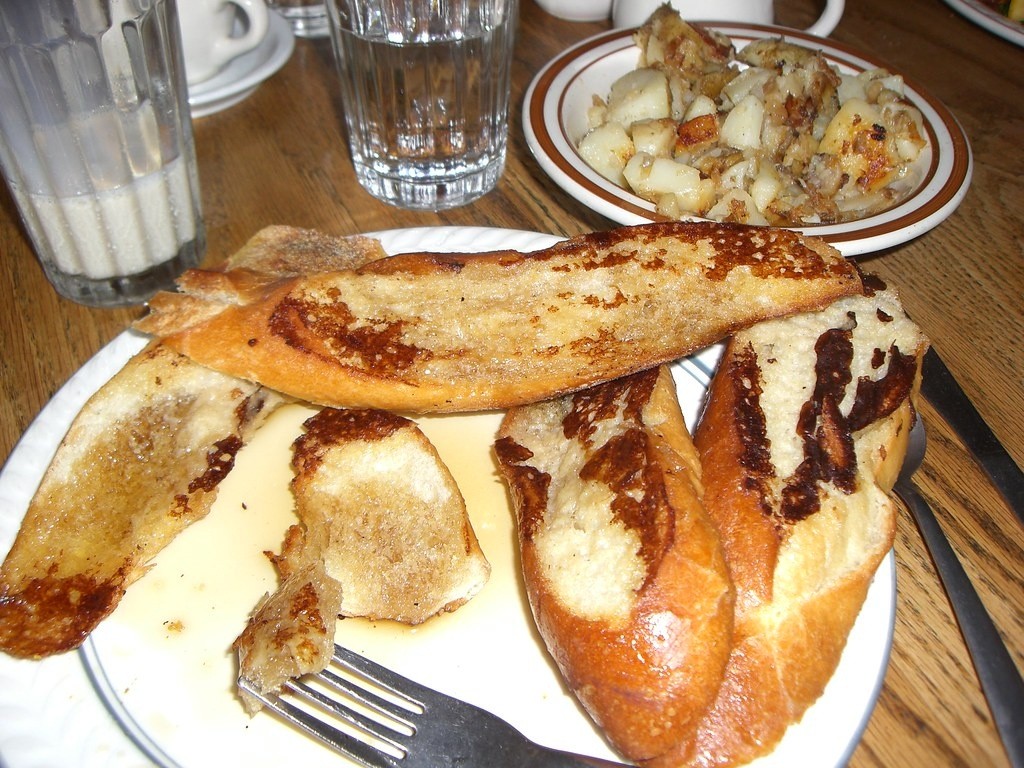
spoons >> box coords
[891,413,1024,768]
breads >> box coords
[1,222,930,768]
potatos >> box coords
[575,63,930,226]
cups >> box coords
[323,0,514,213]
[614,0,845,38]
[0,0,209,310]
[177,0,270,88]
[535,0,613,22]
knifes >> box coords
[921,345,1024,524]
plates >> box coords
[188,8,296,119]
[0,225,898,768]
[521,19,974,255]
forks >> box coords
[237,644,631,768]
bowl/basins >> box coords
[283,4,330,39]
